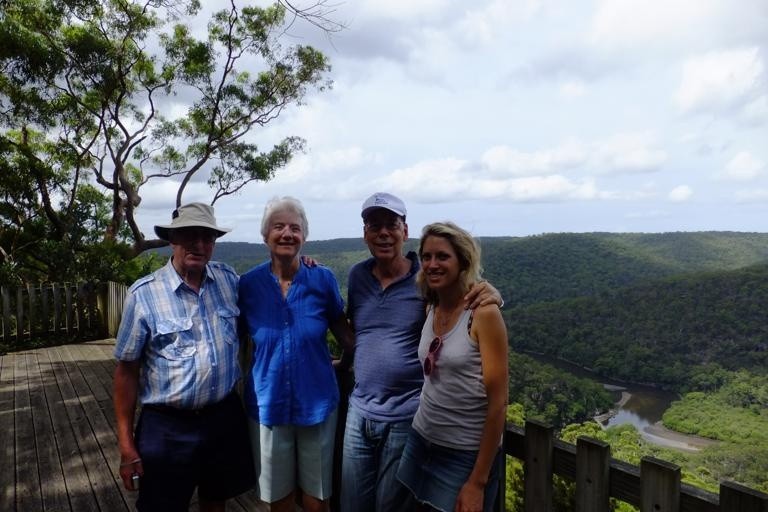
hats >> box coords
[360,191,409,220]
[151,201,234,244]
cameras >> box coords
[129,471,141,490]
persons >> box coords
[111,201,319,511]
[385,222,511,509]
[235,197,353,510]
[338,191,505,510]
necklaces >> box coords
[433,301,458,329]
[275,270,295,290]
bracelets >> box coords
[498,298,505,310]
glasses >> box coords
[365,221,406,231]
[422,336,443,378]
